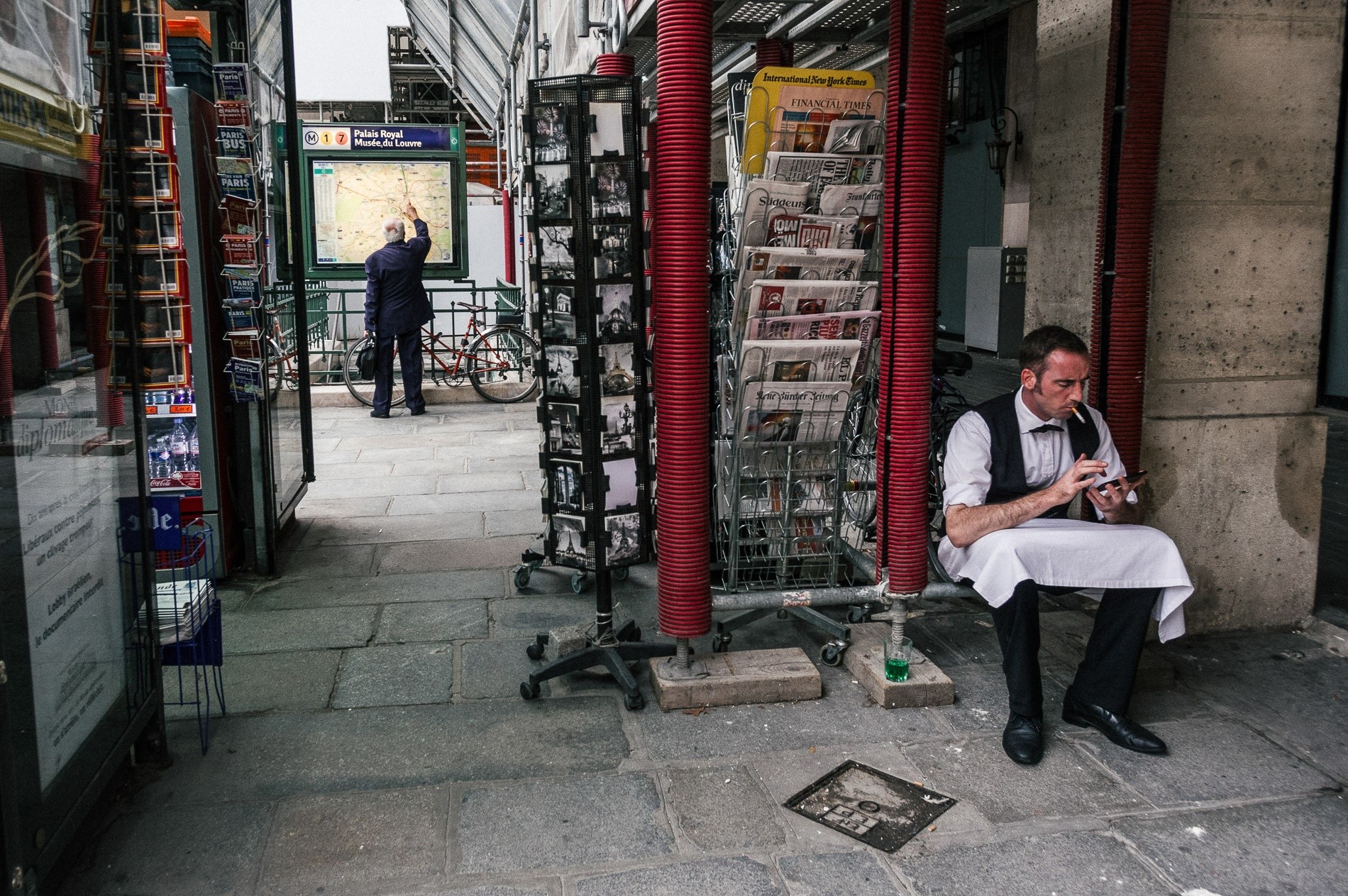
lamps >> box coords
[985,106,1021,174]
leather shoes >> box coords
[1002,708,1044,766]
[1062,685,1167,754]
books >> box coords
[213,62,252,103]
[216,157,257,202]
[231,357,265,401]
[217,126,252,160]
[228,330,261,361]
[216,100,253,139]
[220,193,257,235]
[224,264,260,303]
[224,298,260,334]
[222,235,257,270]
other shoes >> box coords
[411,407,425,416]
[370,410,390,418]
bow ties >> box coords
[1029,422,1065,433]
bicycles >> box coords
[835,308,982,591]
[341,301,543,407]
[265,305,291,403]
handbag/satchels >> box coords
[355,336,377,381]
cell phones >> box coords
[1097,471,1148,492]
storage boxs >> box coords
[166,19,215,103]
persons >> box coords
[937,324,1195,769]
[365,203,436,420]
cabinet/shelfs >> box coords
[211,41,278,402]
[81,0,195,394]
[514,74,692,711]
[716,80,895,665]
